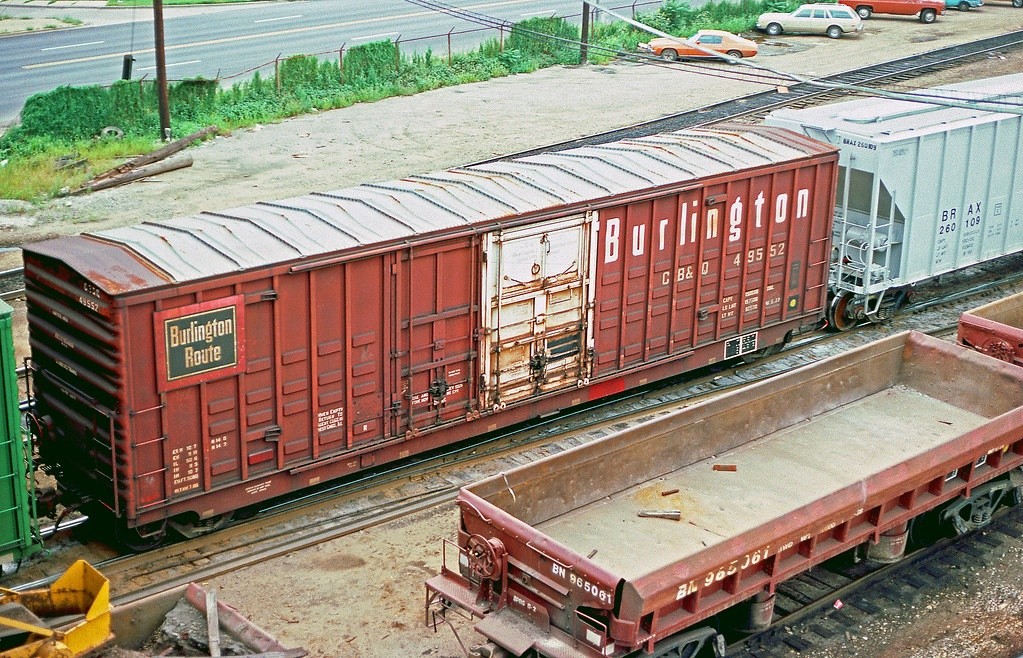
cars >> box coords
[648,29,757,65]
[757,2,863,38]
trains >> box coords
[1,70,1021,565]
[1,290,1023,658]
